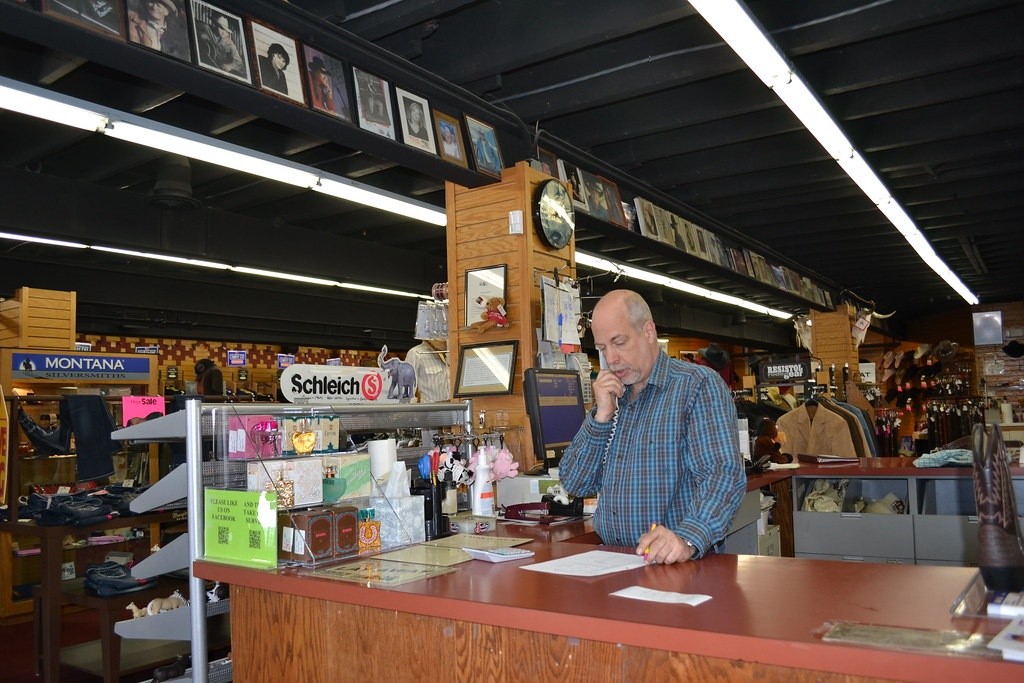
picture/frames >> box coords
[597,175,630,230]
[537,145,559,179]
[39,0,256,90]
[464,264,508,328]
[432,109,470,171]
[246,15,308,108]
[462,111,506,180]
[300,41,356,130]
[395,85,437,154]
[452,340,518,397]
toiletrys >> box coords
[472,445,496,519]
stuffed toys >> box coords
[470,297,509,333]
[427,445,519,482]
[126,585,226,619]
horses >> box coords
[151,653,192,683]
[147,592,183,617]
[126,602,147,619]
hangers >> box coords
[799,383,838,408]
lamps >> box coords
[0,74,108,136]
[578,0,981,319]
[317,170,446,229]
[108,102,315,195]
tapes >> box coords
[554,495,569,505]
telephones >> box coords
[567,353,593,405]
[537,352,567,369]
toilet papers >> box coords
[367,438,397,495]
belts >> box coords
[704,535,725,553]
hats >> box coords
[700,344,729,368]
[1002,340,1024,357]
[880,339,957,379]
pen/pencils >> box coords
[644,521,656,561]
[430,470,437,485]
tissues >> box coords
[369,461,426,547]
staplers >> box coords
[504,502,554,524]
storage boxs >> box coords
[760,524,782,556]
[315,416,339,449]
[331,447,371,499]
[332,506,359,556]
[247,460,283,491]
[295,511,334,561]
[282,417,302,454]
[236,415,282,457]
[229,415,237,457]
[758,512,772,534]
[451,515,497,533]
[726,488,762,537]
[284,461,324,505]
[725,523,759,555]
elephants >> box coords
[378,345,417,399]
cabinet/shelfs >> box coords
[0,344,163,627]
[110,400,192,683]
[791,457,1024,589]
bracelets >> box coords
[686,540,692,547]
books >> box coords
[987,591,1024,662]
[539,276,581,345]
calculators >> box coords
[461,547,536,563]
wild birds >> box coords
[194,360,224,403]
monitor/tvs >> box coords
[522,368,586,469]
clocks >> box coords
[533,179,577,250]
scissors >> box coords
[417,454,431,480]
[429,452,439,474]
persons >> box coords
[542,160,551,175]
[685,223,695,251]
[716,239,727,265]
[407,103,428,141]
[405,342,450,445]
[568,174,580,199]
[476,129,498,169]
[307,56,335,112]
[129,0,178,56]
[368,80,383,116]
[443,127,458,158]
[645,208,657,236]
[259,43,290,95]
[759,259,769,280]
[586,179,608,217]
[670,215,686,251]
[558,291,747,565]
[196,13,243,77]
[39,415,56,434]
